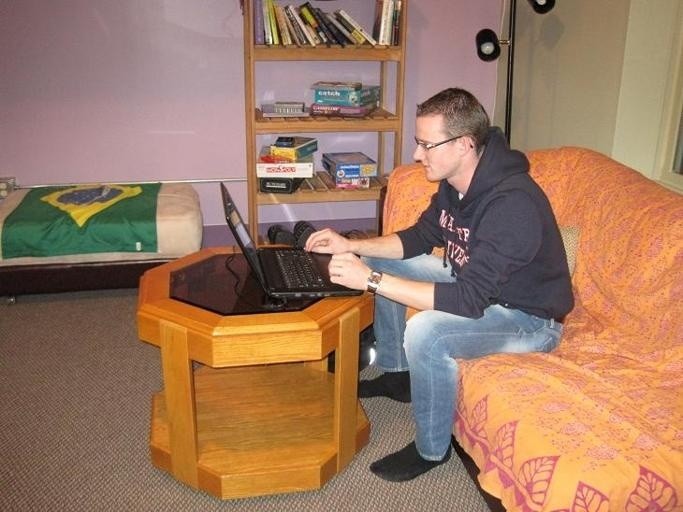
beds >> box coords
[0,178,205,306]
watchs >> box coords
[366,269,382,294]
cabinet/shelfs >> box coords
[241,0,410,251]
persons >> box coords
[300,88,574,483]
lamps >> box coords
[476,0,557,149]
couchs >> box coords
[378,144,683,510]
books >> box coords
[249,1,404,120]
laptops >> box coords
[220,181,364,299]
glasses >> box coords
[414,136,462,152]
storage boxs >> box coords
[257,136,377,194]
